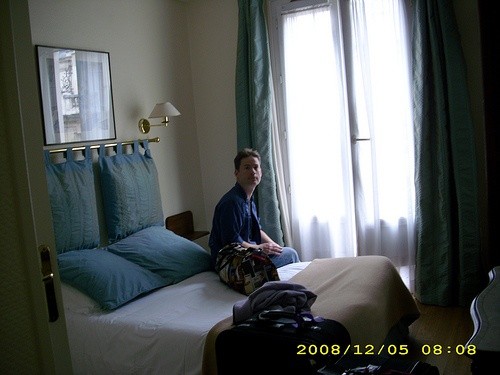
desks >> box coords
[462,263,500,375]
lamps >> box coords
[138,101,182,135]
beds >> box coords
[56,255,411,375]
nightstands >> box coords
[164,208,211,253]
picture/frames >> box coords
[34,44,117,147]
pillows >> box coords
[56,224,210,313]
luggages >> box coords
[214,306,352,375]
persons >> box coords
[208,149,301,270]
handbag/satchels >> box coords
[212,242,280,296]
